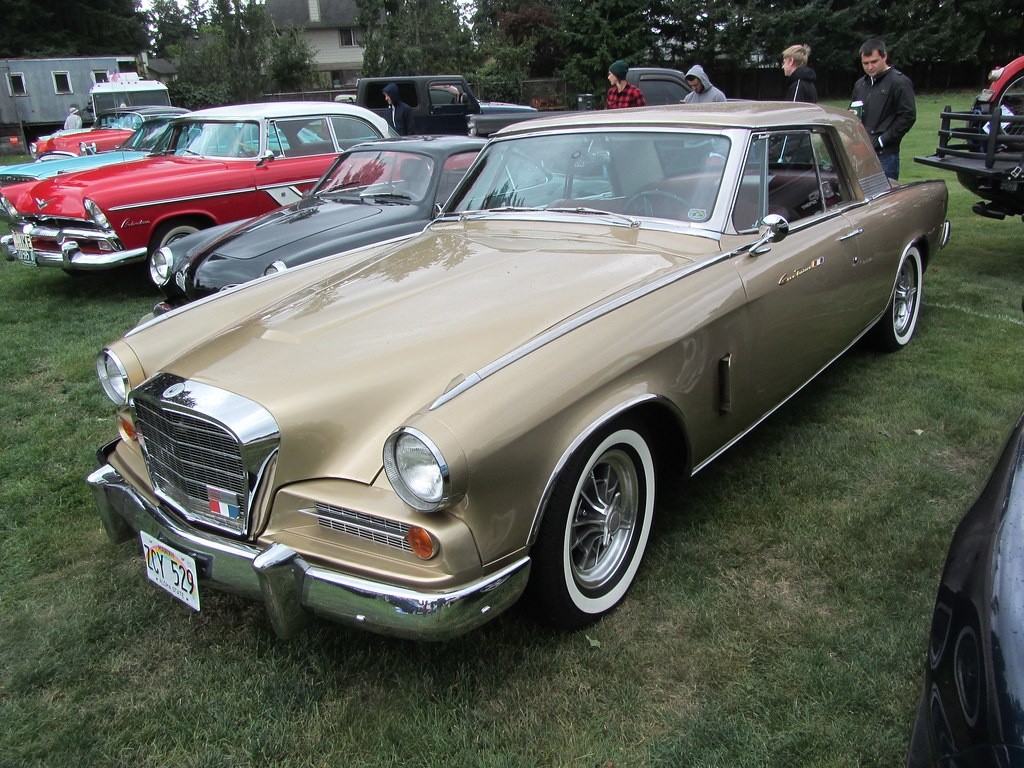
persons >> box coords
[774,45,818,163]
[603,60,645,109]
[64,107,82,130]
[847,38,916,181]
[683,65,727,103]
[382,83,415,136]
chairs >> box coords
[394,159,431,197]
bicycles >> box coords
[976,57,1024,152]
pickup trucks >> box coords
[911,56,1024,227]
[465,64,694,179]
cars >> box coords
[1,72,538,224]
[907,414,1022,767]
[89,99,953,643]
[148,131,611,322]
[0,102,483,296]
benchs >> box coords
[297,139,368,156]
[545,171,841,229]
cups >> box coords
[851,101,863,120]
